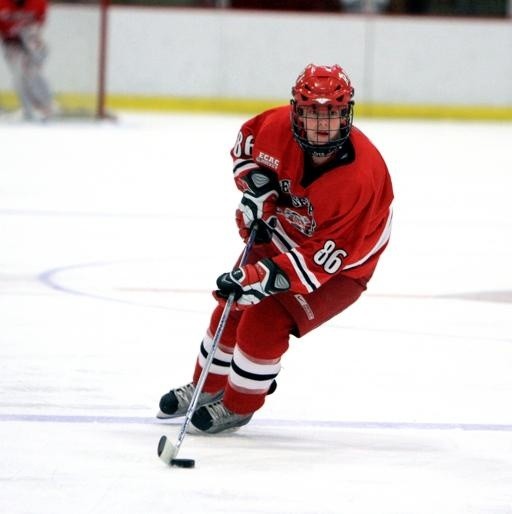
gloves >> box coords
[212,257,290,312]
[235,170,280,246]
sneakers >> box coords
[159,382,224,415]
[190,398,254,433]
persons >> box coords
[0,1,59,124]
[159,61,397,436]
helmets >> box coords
[290,63,355,156]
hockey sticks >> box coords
[158,226,258,464]
